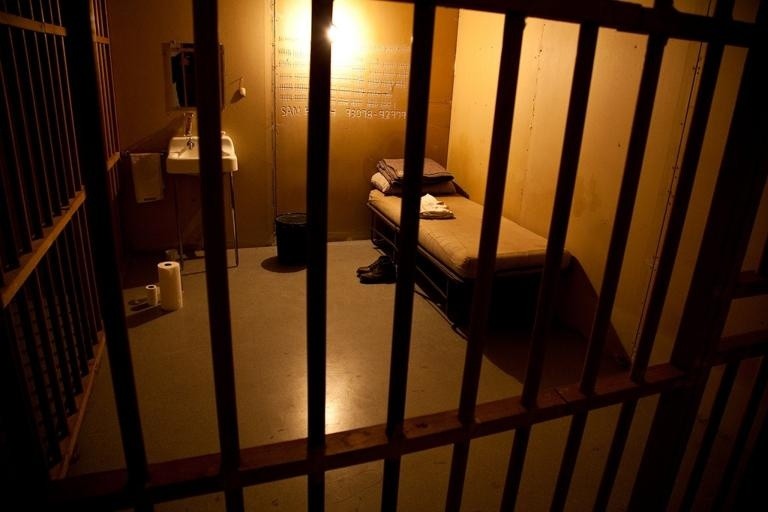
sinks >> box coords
[165,136,239,176]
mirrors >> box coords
[160,38,224,114]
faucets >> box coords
[187,137,196,149]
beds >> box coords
[365,188,573,342]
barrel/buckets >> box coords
[273,212,307,267]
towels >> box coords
[129,152,166,205]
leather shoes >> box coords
[357,256,395,284]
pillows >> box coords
[366,171,459,197]
